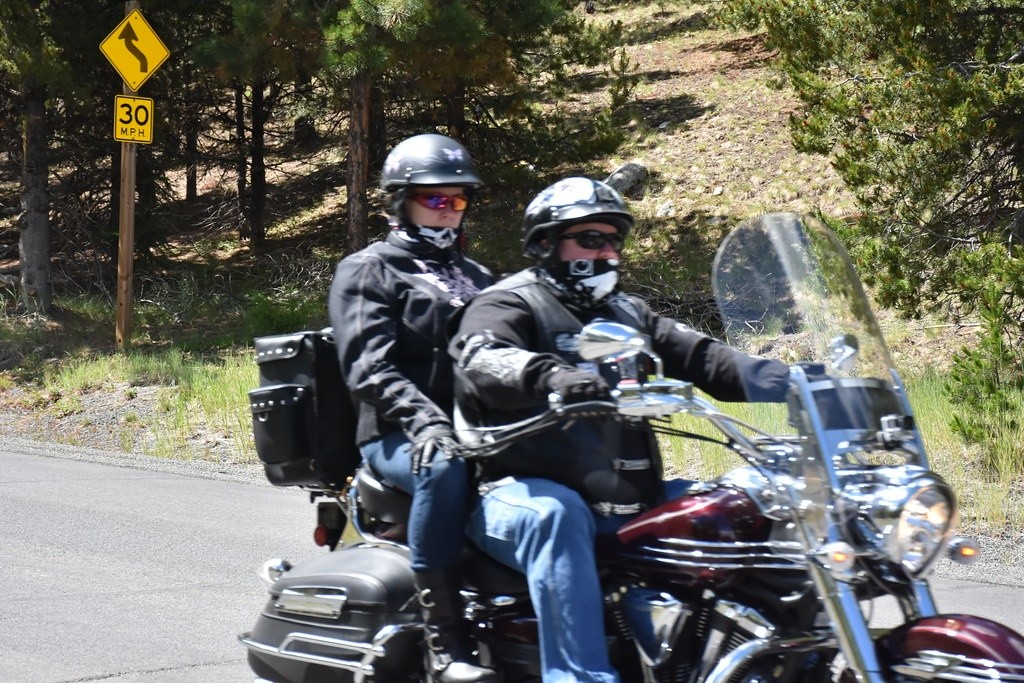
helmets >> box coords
[519,176,636,269]
[377,133,485,232]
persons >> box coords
[449,175,826,683]
[328,132,509,683]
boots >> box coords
[413,572,495,681]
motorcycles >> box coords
[237,211,1022,682]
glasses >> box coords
[552,227,627,253]
[406,190,469,211]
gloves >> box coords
[547,366,612,419]
[791,362,835,384]
[407,423,465,474]
